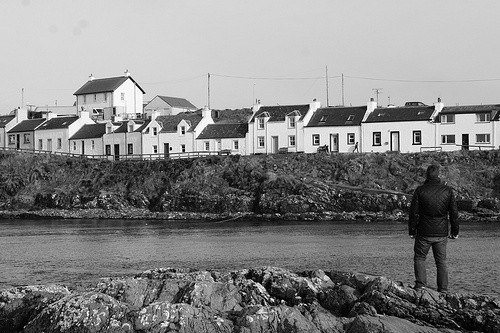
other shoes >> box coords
[437,289,449,295]
[413,286,425,292]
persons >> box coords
[352,142,359,153]
[319,145,327,153]
[408,164,460,293]
